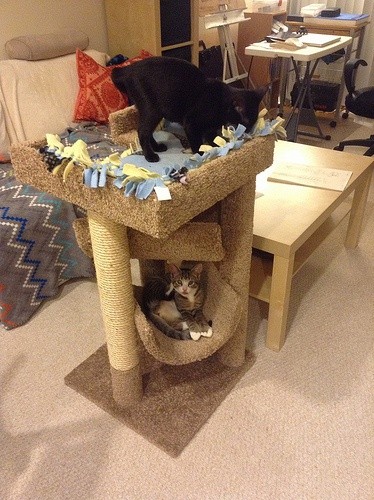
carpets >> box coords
[264,106,363,149]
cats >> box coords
[110,57,272,162]
[143,262,213,341]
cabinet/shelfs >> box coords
[277,12,372,128]
[236,7,288,111]
[104,0,199,71]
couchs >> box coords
[0,28,94,330]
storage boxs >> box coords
[291,79,341,112]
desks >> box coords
[10,127,275,459]
[244,32,353,142]
[165,138,374,352]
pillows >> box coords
[71,45,155,126]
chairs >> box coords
[333,58,374,155]
